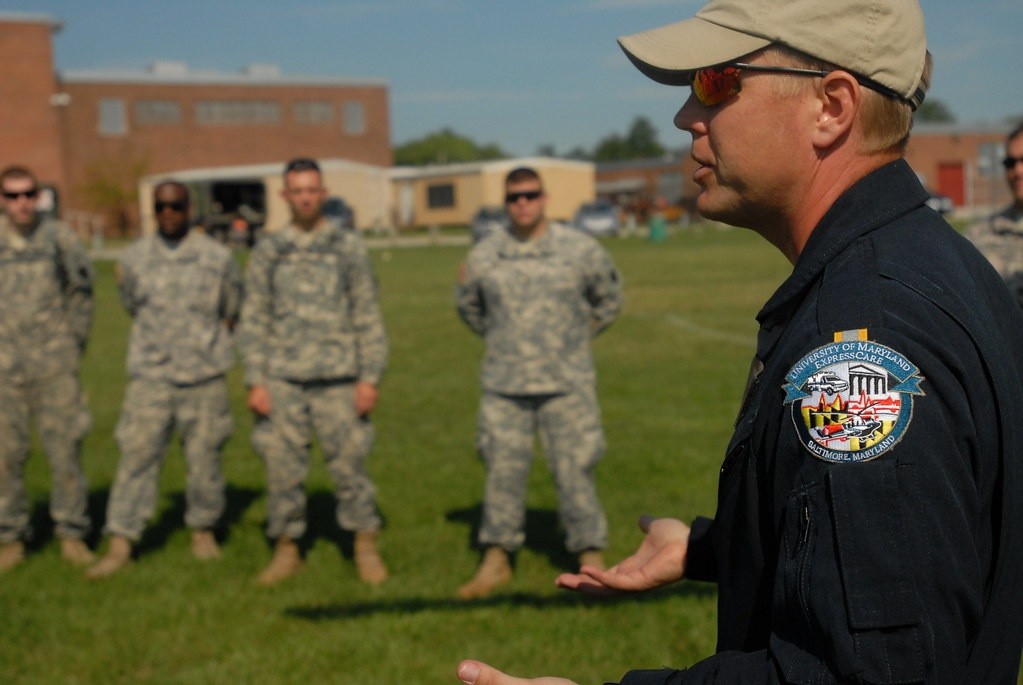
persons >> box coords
[456,0,1023,685]
[240,156,389,586]
[0,167,94,572]
[85,178,240,578]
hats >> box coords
[617,0,927,113]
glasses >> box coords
[690,60,831,106]
[506,189,543,203]
[0,186,38,199]
[155,198,187,213]
[1002,155,1023,170]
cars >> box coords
[574,199,621,239]
[471,206,509,241]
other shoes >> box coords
[190,527,219,559]
[579,548,605,572]
[456,545,512,600]
[353,528,388,584]
[261,535,300,584]
[0,542,26,571]
[61,535,96,566]
[86,535,132,578]
